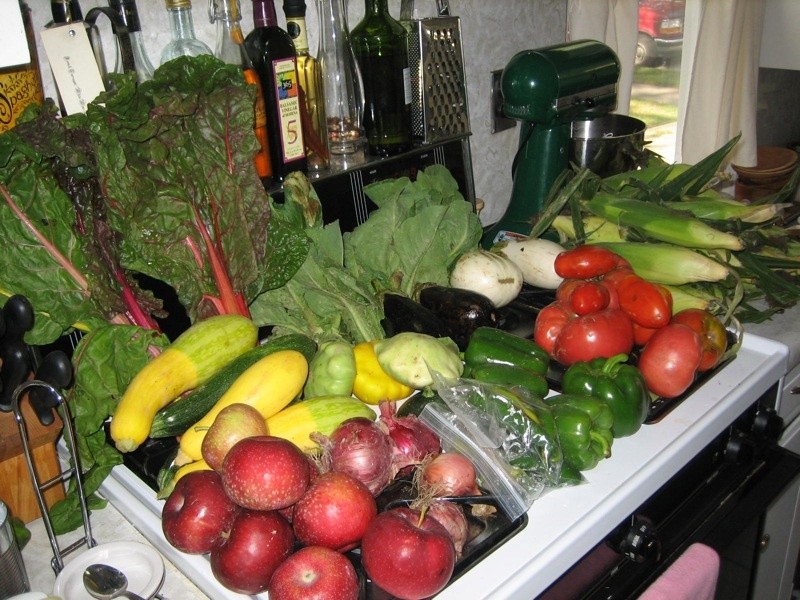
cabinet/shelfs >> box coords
[273,139,470,235]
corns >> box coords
[547,160,800,315]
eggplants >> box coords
[378,238,567,349]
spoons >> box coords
[82,564,145,600]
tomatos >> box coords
[531,246,727,399]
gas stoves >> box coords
[58,132,787,597]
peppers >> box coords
[461,327,649,488]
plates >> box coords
[52,540,166,600]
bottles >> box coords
[313,1,362,156]
[281,1,331,171]
[0,500,31,600]
[348,1,420,161]
[158,1,214,68]
[47,0,110,116]
[203,0,272,192]
[106,0,155,91]
[243,0,308,191]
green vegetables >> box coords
[251,164,484,341]
[0,53,313,535]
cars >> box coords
[635,0,684,67]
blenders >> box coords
[480,40,646,258]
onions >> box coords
[313,412,482,557]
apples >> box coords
[161,405,456,600]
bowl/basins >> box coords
[571,116,648,179]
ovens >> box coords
[549,384,800,599]
